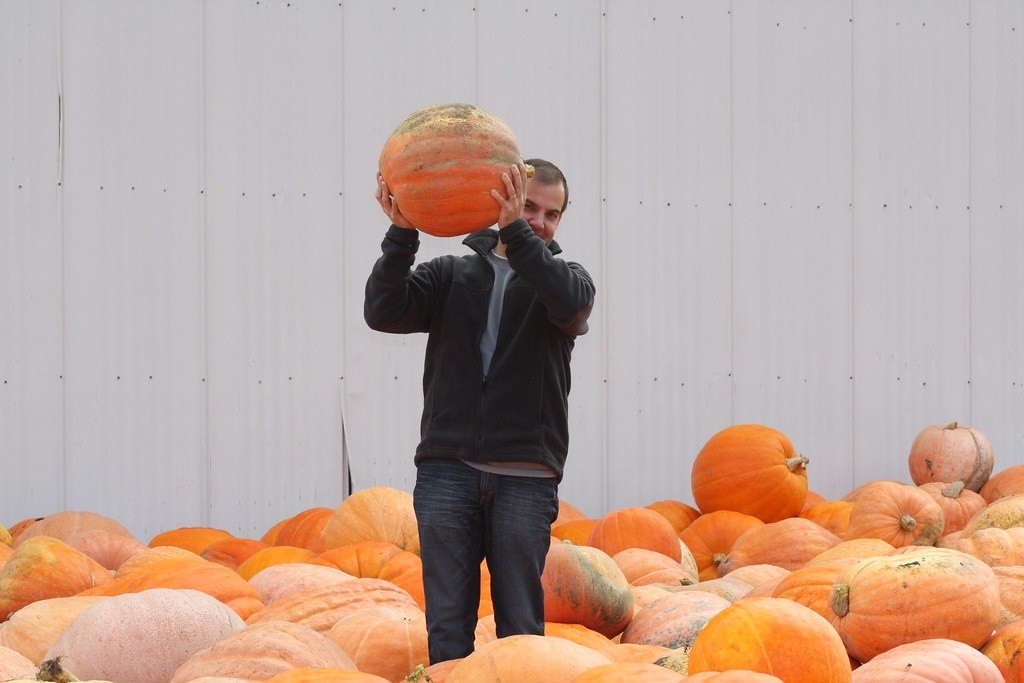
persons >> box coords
[359,156,595,667]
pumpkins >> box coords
[0,422,1024,682]
[378,103,535,239]
